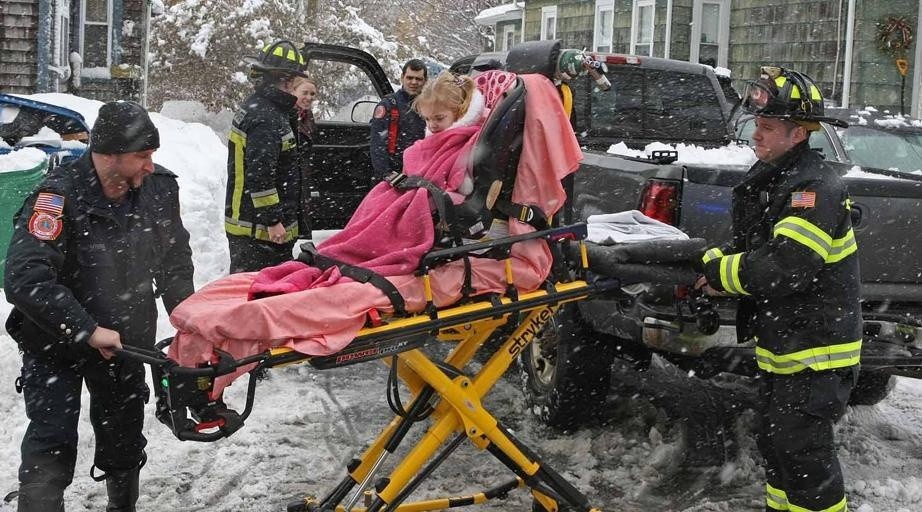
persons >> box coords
[4,99,196,512]
[281,73,318,239]
[224,38,310,272]
[413,71,493,135]
[691,66,863,511]
[369,59,428,183]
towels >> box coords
[582,209,692,248]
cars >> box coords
[733,108,920,163]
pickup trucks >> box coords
[304,43,921,426]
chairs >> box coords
[458,71,527,238]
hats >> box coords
[88,99,159,156]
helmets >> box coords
[246,39,307,86]
[740,67,851,129]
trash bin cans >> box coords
[0,94,89,169]
[0,155,49,288]
